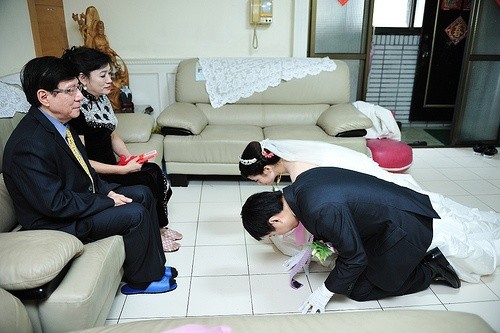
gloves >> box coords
[299,282,334,315]
[282,245,312,275]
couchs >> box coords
[157,57,372,187]
[0,72,165,333]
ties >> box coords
[289,247,313,290]
[65,128,96,194]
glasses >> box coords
[53,84,83,96]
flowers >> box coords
[309,240,336,263]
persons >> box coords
[61,46,182,253]
[92,21,121,68]
[1,55,179,294]
[239,139,500,285]
[241,167,460,314]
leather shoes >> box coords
[424,247,461,289]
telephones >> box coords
[250,0,273,25]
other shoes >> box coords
[473,142,498,158]
[407,141,427,147]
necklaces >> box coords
[277,160,284,183]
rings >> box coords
[308,304,311,306]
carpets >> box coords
[400,127,445,145]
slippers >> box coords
[122,266,180,295]
[159,226,183,252]
